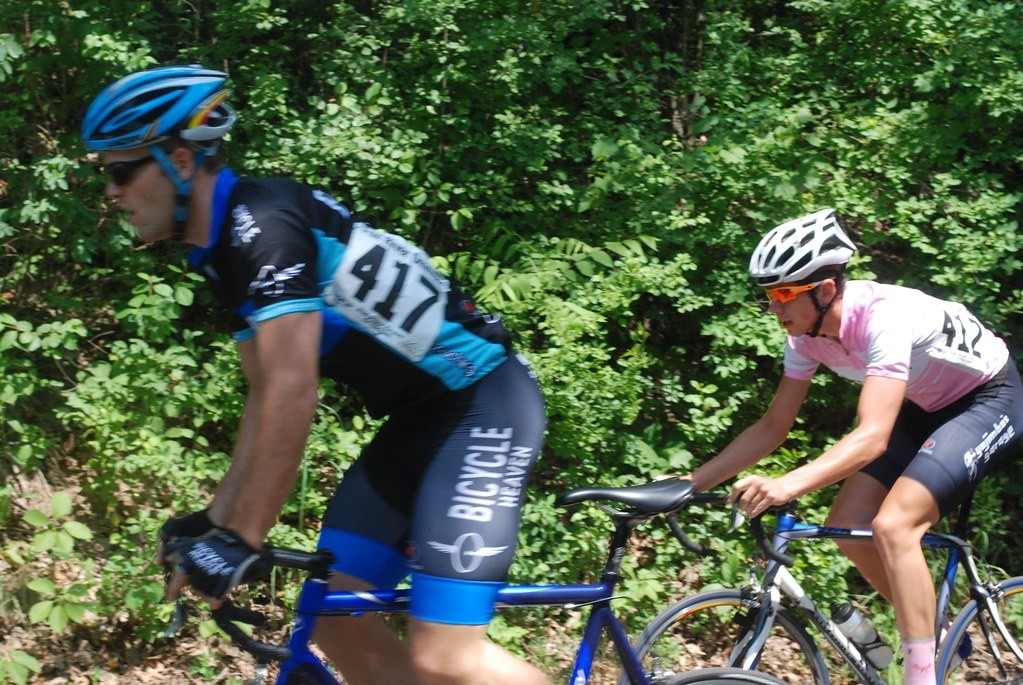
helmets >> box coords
[80,63,237,155]
[749,208,855,286]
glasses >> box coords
[766,277,836,304]
[91,151,173,186]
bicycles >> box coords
[161,478,786,685]
[615,483,1022,685]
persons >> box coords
[77,65,555,685]
[653,209,1022,685]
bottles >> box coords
[828,601,894,672]
